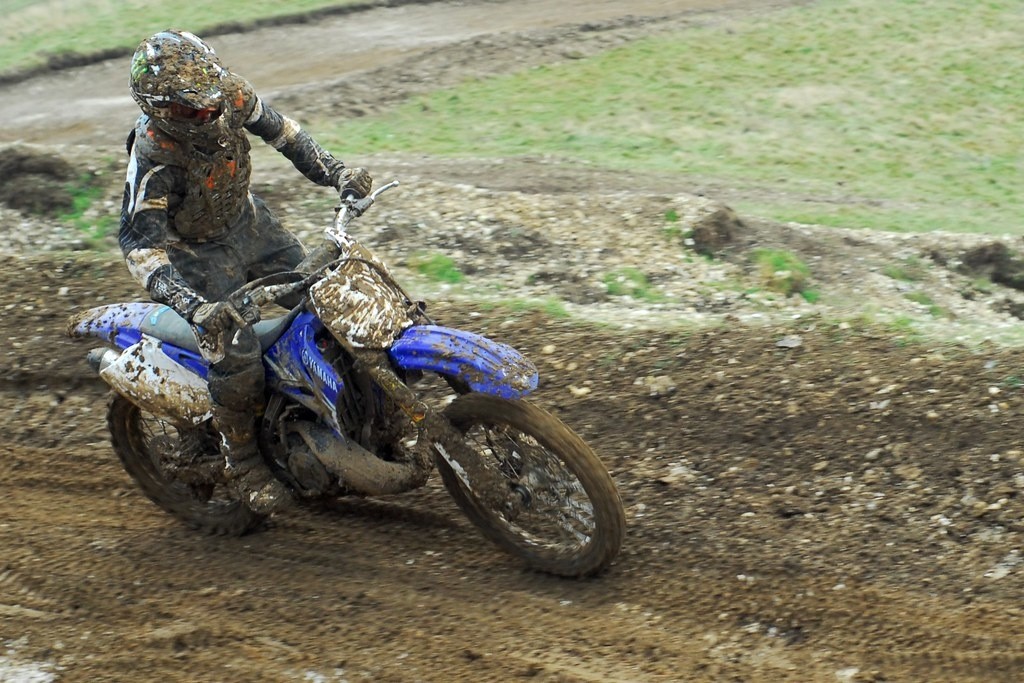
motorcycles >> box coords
[70,180,625,579]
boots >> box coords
[211,400,290,515]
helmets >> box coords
[128,27,237,159]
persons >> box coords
[118,29,373,515]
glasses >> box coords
[170,102,219,123]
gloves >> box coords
[333,167,373,198]
[190,301,246,337]
